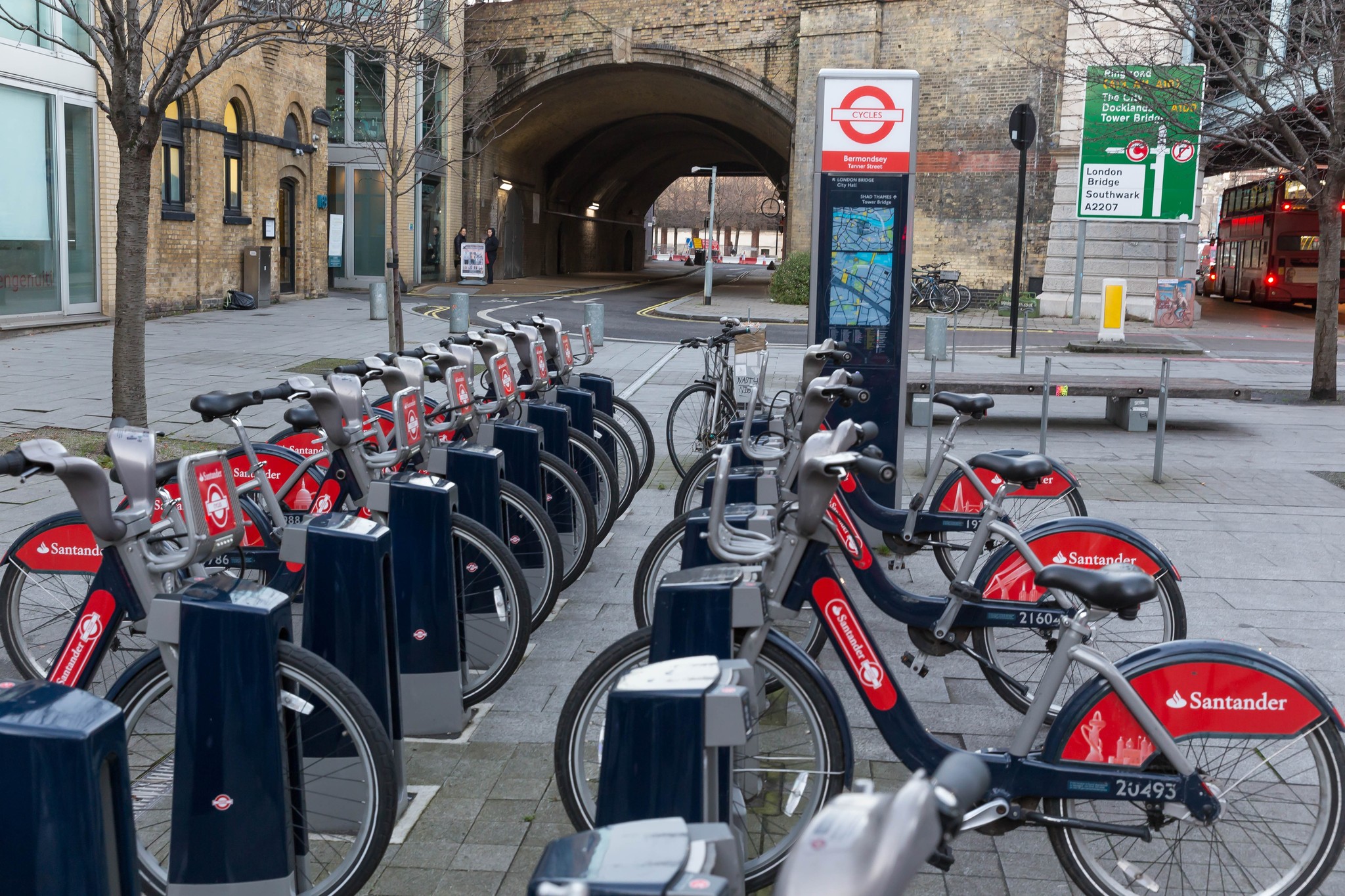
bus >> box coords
[1213,165,1345,311]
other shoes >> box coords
[454,259,458,268]
[487,281,494,284]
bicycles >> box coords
[551,422,1339,896]
[761,183,787,217]
[911,262,972,312]
[1,360,524,740]
[110,353,574,638]
[634,420,1193,728]
[0,435,399,894]
[672,339,1089,595]
[269,309,664,597]
[664,317,749,475]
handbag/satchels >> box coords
[485,252,489,264]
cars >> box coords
[1195,264,1216,298]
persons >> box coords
[462,242,483,274]
[429,226,441,276]
[453,227,468,269]
[482,227,499,284]
[730,241,733,256]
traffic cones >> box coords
[762,252,766,265]
[740,251,746,265]
[684,251,688,261]
[667,250,674,262]
[653,246,658,260]
[717,252,722,263]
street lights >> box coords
[691,164,714,304]
